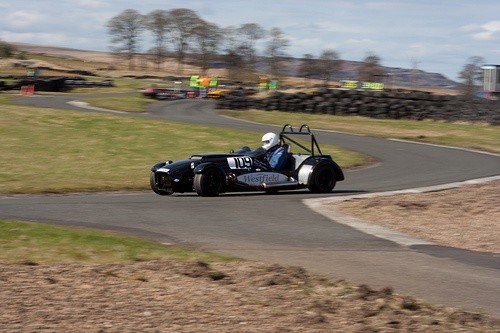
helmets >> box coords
[262,132,279,150]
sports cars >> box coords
[150,124,345,198]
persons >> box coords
[261,131,289,170]
